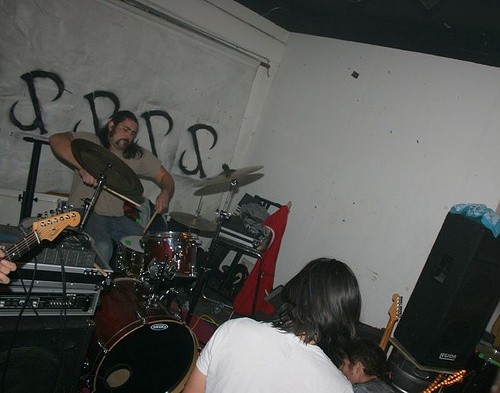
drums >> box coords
[81,278,202,393]
[462,329,499,393]
[142,231,198,278]
[115,235,147,274]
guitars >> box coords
[378,293,404,350]
[0,208,81,267]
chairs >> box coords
[202,196,288,306]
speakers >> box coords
[0,316,95,393]
[394,211,500,367]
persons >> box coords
[182,257,362,393]
[50,110,174,264]
[0,249,17,284]
[339,338,395,393]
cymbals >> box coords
[70,138,144,200]
[170,212,218,232]
[193,165,265,188]
